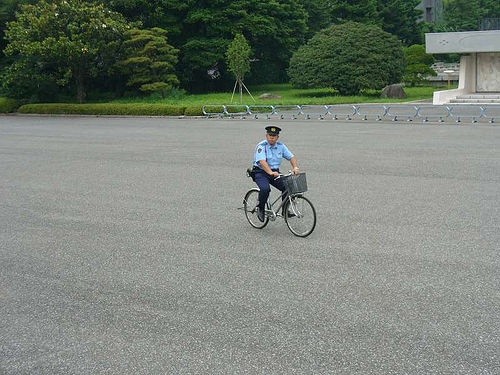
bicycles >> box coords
[237,166,317,238]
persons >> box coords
[253,126,300,222]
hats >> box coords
[265,126,281,136]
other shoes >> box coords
[257,205,265,222]
[282,212,295,217]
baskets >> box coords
[280,173,307,194]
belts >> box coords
[252,169,279,172]
